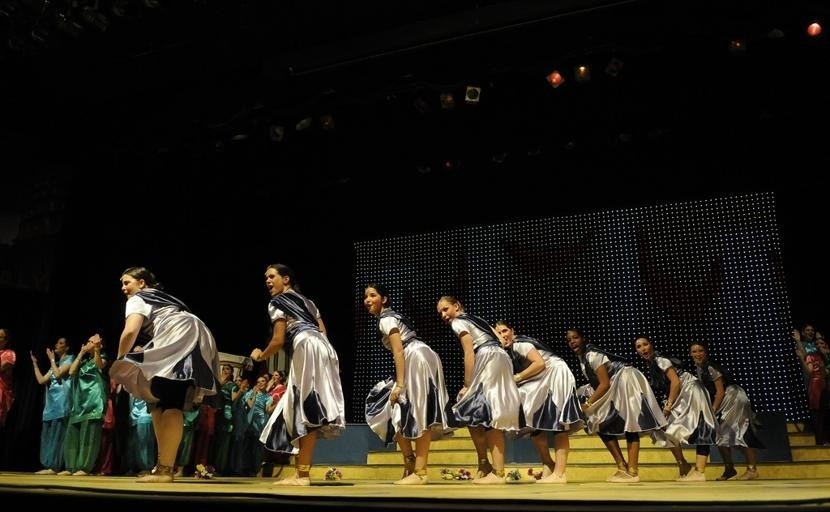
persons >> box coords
[249,263,346,488]
[109,266,220,482]
[790,322,824,433]
[633,335,724,483]
[435,295,530,483]
[796,335,830,446]
[689,340,768,481]
[360,282,459,484]
[495,319,589,483]
[566,327,668,485]
[0,317,289,478]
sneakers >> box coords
[677,465,706,482]
[57,470,71,475]
[536,462,567,483]
[136,474,172,483]
[738,469,759,480]
[73,470,87,476]
[716,470,737,480]
[35,469,54,474]
[393,457,427,485]
[611,470,639,484]
[474,466,505,484]
[274,471,311,485]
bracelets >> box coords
[665,401,672,408]
[396,382,406,388]
[712,406,717,412]
[463,380,472,388]
[583,399,591,409]
[514,372,522,382]
